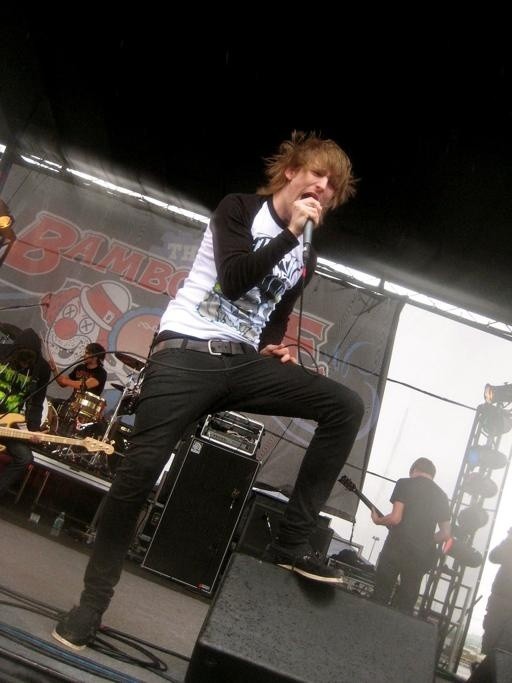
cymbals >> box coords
[111,383,141,394]
[115,351,146,370]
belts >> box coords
[150,337,246,356]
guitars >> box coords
[337,474,392,529]
[0,412,116,455]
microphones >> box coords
[44,295,53,318]
[303,218,314,261]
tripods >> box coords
[52,377,133,478]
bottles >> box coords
[50,510,67,530]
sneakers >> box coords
[87,343,105,361]
[272,548,345,582]
[52,604,102,650]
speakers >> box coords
[183,551,440,683]
[465,647,512,683]
[140,436,262,598]
[236,503,334,556]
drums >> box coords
[39,401,59,434]
[67,388,109,421]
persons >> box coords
[51,129,365,650]
[370,456,455,615]
[47,342,108,440]
[469,525,511,672]
[0,326,53,497]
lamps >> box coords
[0,200,15,232]
[444,381,511,570]
